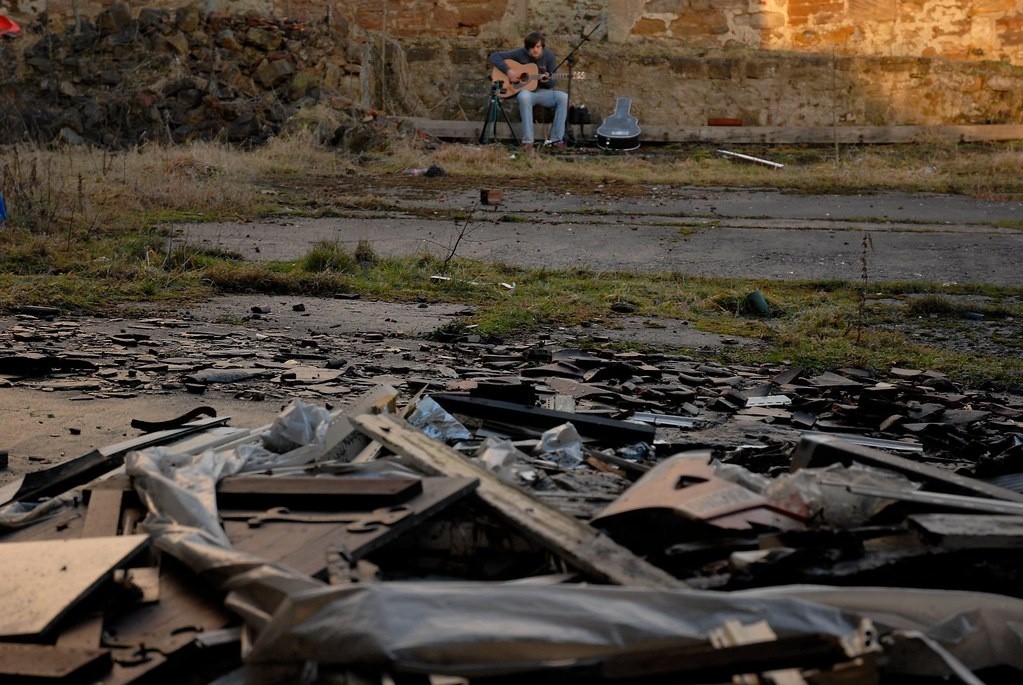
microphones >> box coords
[542,76,548,81]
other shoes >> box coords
[553,141,566,146]
[522,143,534,149]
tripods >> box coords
[480,80,523,148]
[544,23,601,153]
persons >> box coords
[489,31,568,149]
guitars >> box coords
[491,59,587,99]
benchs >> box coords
[479,108,603,146]
